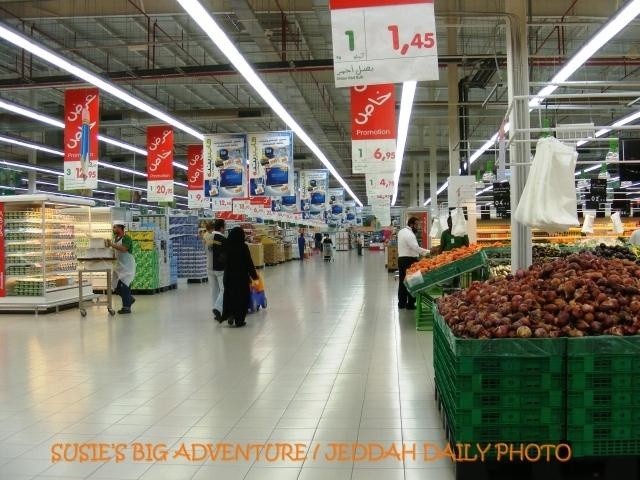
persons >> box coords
[356,233,364,256]
[298,233,306,262]
[397,217,432,310]
[439,216,469,295]
[201,219,259,328]
[320,233,334,262]
[104,219,136,314]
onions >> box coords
[435,254,640,338]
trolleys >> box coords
[323,243,334,263]
[79,269,117,315]
[247,289,269,312]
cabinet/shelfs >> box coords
[0,193,316,314]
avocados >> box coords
[489,243,640,263]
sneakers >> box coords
[399,303,416,310]
[118,297,136,314]
[212,309,246,327]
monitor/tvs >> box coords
[205,167,361,224]
